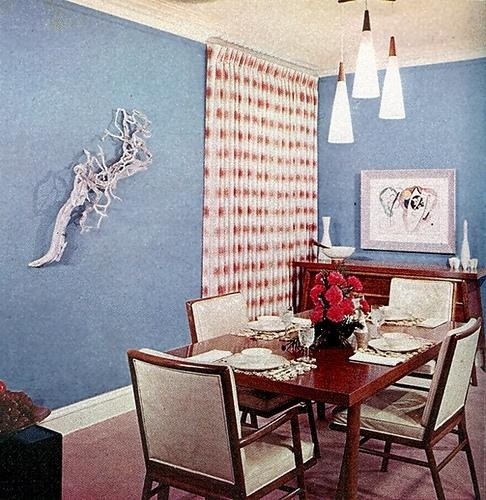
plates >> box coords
[371,311,409,321]
[245,320,285,332]
[368,338,421,352]
[227,352,281,372]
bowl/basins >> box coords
[321,246,357,261]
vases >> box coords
[319,217,331,260]
[460,220,470,270]
[314,322,356,346]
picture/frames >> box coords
[361,170,456,254]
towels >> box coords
[292,317,310,326]
[420,318,447,327]
[349,352,404,366]
[187,350,231,362]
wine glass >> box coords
[296,327,317,363]
[449,257,478,273]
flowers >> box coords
[311,270,369,321]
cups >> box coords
[256,315,281,328]
[377,306,402,318]
[382,331,416,349]
[240,347,273,366]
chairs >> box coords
[126,347,318,500]
[329,317,482,500]
[185,291,301,428]
[387,277,459,392]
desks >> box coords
[294,261,486,350]
[0,424,62,499]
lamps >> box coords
[378,0,406,120]
[352,0,381,99]
[326,0,354,144]
